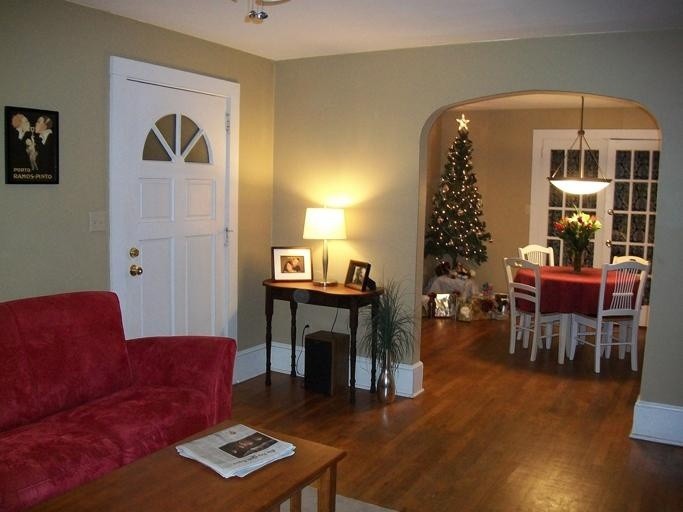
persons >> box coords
[10,113,31,167]
[283,257,300,271]
[26,135,39,171]
[283,261,297,273]
[35,114,53,168]
[356,267,367,284]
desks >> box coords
[511,265,640,367]
[262,278,384,405]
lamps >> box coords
[545,96,613,196]
[301,207,348,287]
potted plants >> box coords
[351,276,417,405]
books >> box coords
[174,423,297,480]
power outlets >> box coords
[301,318,313,337]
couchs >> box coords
[0,291,238,512]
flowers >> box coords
[551,202,601,273]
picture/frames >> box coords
[4,106,60,185]
[270,245,313,284]
[343,260,376,293]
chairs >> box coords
[502,257,559,363]
[515,244,561,350]
[567,260,650,375]
[578,254,647,361]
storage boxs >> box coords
[419,261,510,323]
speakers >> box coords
[305,330,350,397]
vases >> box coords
[571,248,583,274]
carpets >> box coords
[278,484,403,512]
